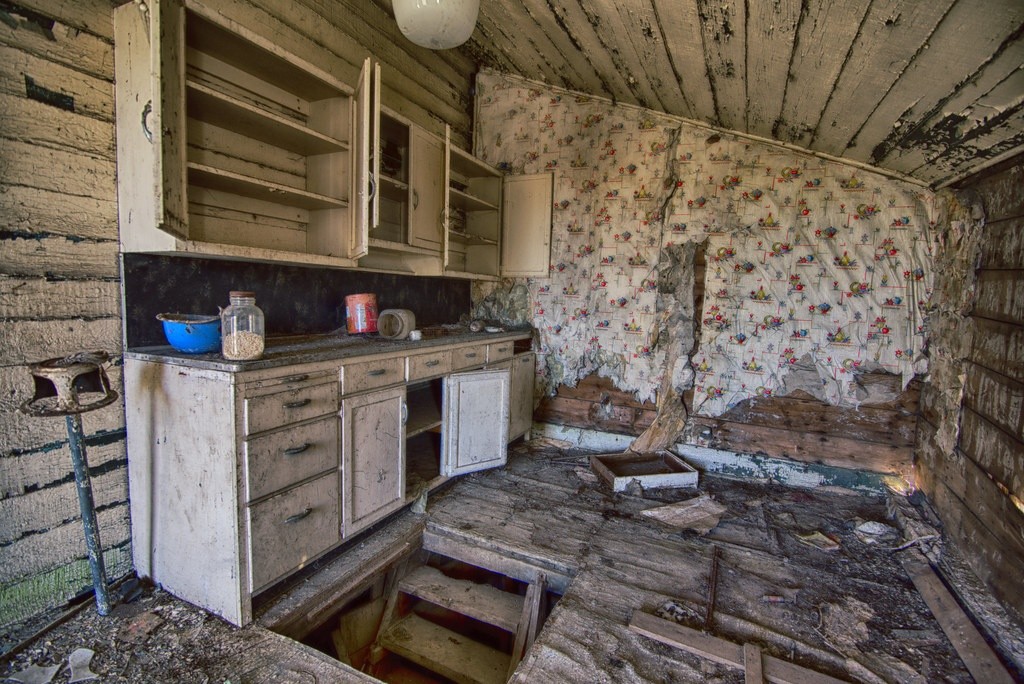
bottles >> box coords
[222,291,265,361]
[409,326,449,341]
[377,309,415,340]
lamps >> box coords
[392,0,480,49]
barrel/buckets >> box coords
[345,293,378,334]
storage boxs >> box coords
[588,450,699,495]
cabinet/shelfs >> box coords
[124,332,537,628]
[113,0,554,284]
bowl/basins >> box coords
[155,313,222,354]
